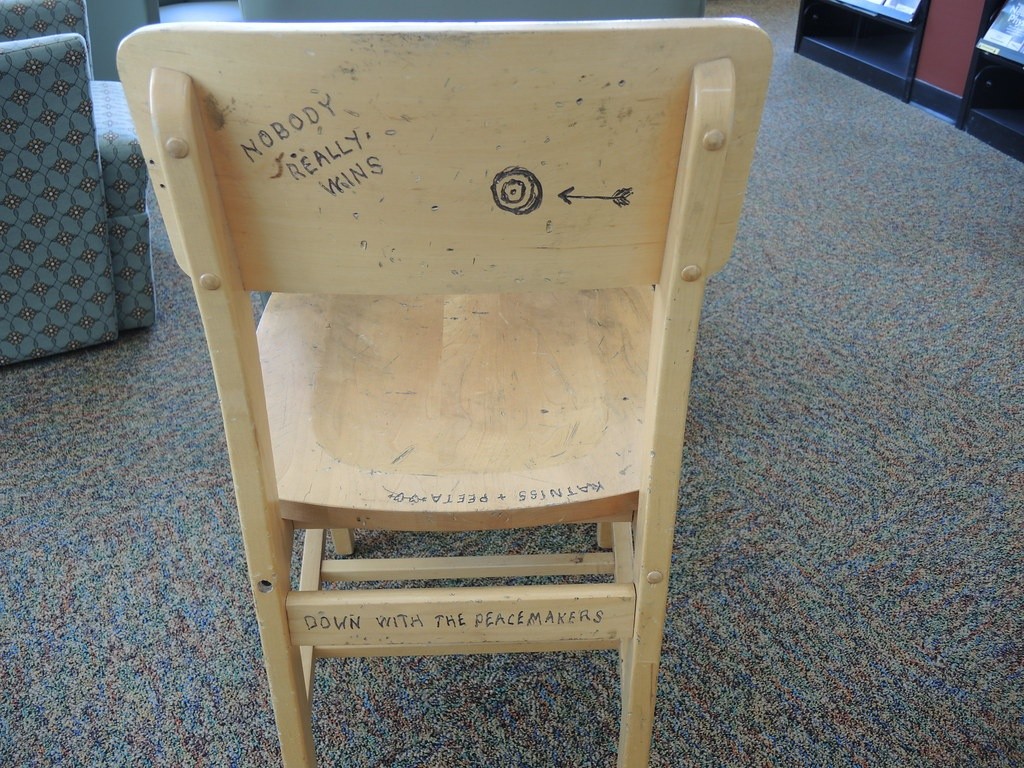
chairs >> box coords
[111,15,775,768]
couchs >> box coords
[0,0,157,372]
